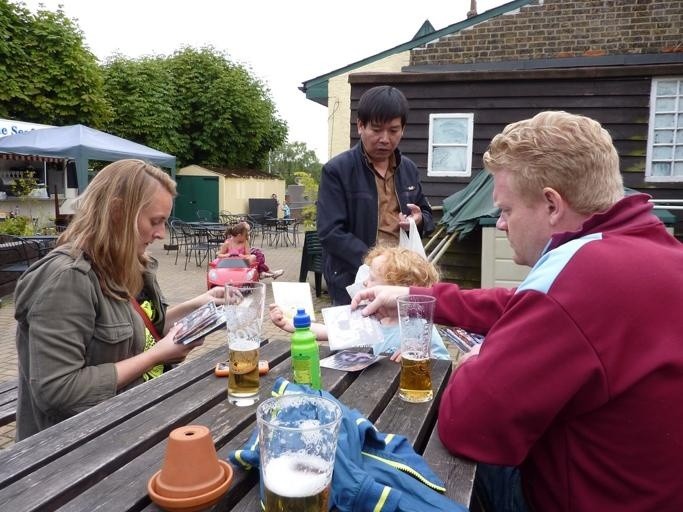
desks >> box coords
[11,236,62,261]
[0,337,481,512]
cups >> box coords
[224,280,266,408]
[394,293,437,404]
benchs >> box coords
[0,379,20,428]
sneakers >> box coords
[207,261,215,268]
[259,269,284,279]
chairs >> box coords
[164,209,304,272]
[0,233,55,274]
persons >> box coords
[280,201,291,225]
[351,110,683,512]
[14,159,244,443]
[268,239,450,363]
[217,223,255,263]
[316,86,435,306]
[241,222,284,280]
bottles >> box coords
[257,393,343,512]
[289,307,321,391]
